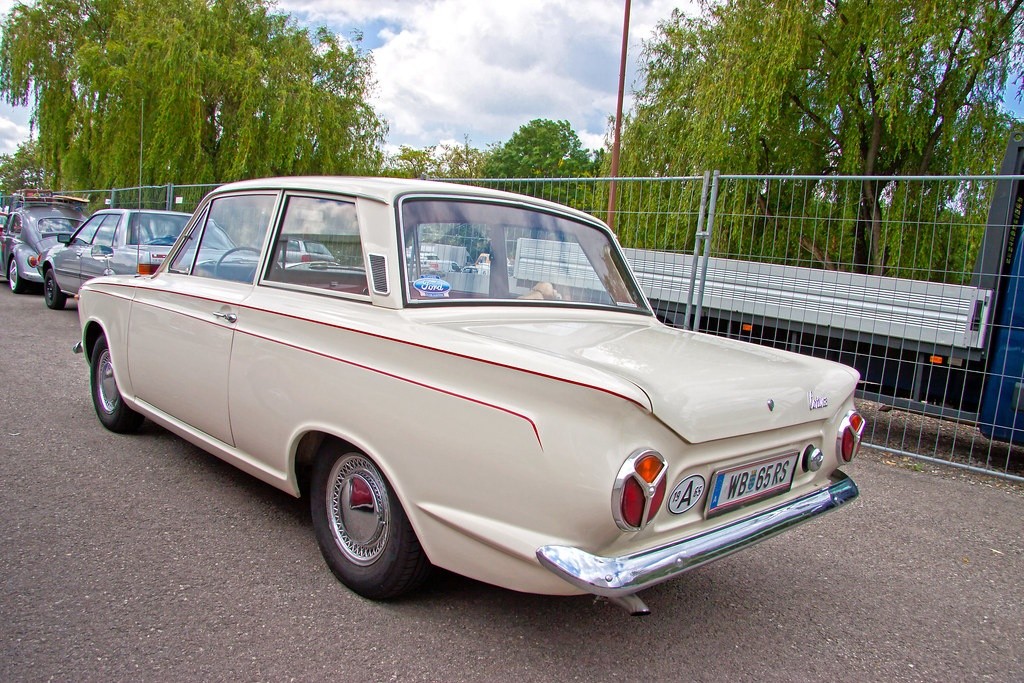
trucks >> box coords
[409,242,476,271]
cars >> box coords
[406,252,514,277]
[75,177,867,617]
[275,239,336,264]
[0,189,94,294]
[43,208,244,314]
